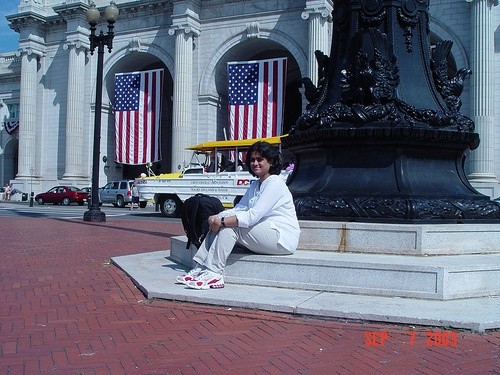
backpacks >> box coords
[180,193,225,250]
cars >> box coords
[80,186,91,198]
[35,185,90,206]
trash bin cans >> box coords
[22,193,28,201]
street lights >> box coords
[83,0,120,222]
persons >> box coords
[175,141,301,289]
[237,160,244,171]
[3,184,12,201]
[207,151,215,173]
[129,181,140,211]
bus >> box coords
[132,133,294,218]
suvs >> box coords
[99,180,135,208]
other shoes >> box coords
[176,269,201,284]
[137,209,140,211]
[186,269,224,289]
[129,208,133,211]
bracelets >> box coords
[221,217,225,227]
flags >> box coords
[226,57,288,164]
[113,68,163,165]
